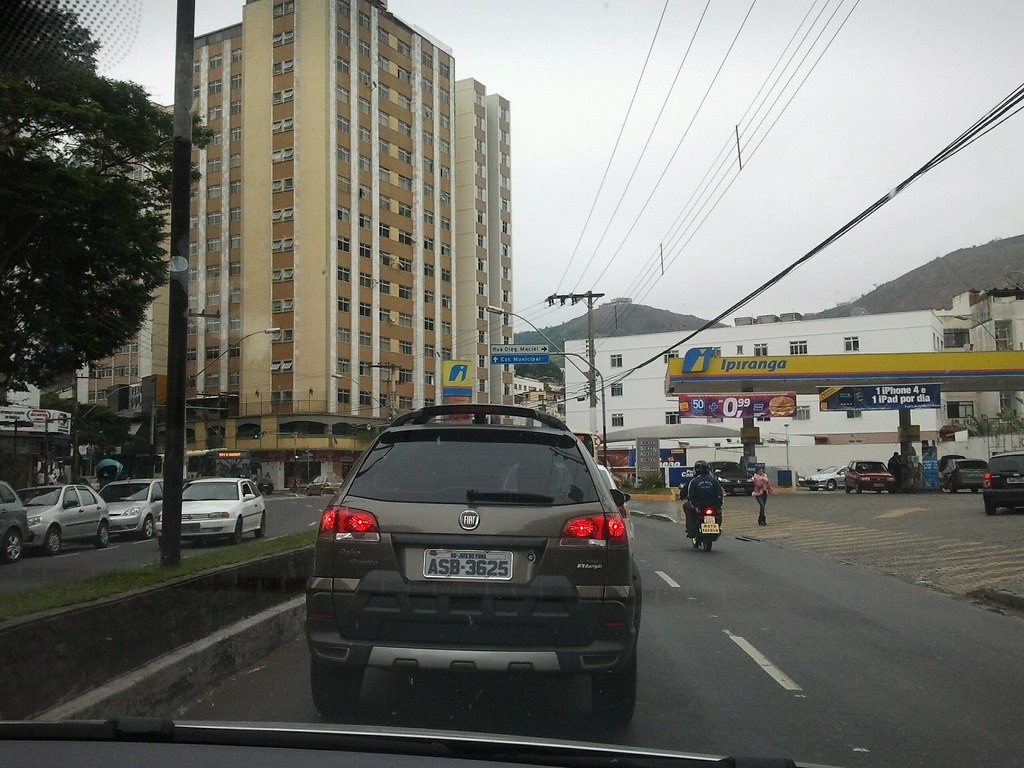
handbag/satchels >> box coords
[767,485,773,492]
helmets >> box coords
[695,460,709,475]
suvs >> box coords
[982,450,1024,515]
[497,457,635,559]
[0,479,29,564]
[305,402,641,723]
[940,460,988,493]
[13,485,110,556]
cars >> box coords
[708,461,755,496]
[305,475,343,496]
[242,473,274,495]
[844,461,897,495]
[804,465,847,490]
[156,477,266,547]
[98,477,162,538]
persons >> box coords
[678,460,724,539]
[751,465,773,527]
[37,467,60,486]
[887,451,906,491]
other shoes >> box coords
[760,516,764,526]
[758,516,766,524]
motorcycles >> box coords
[678,483,723,552]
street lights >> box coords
[44,417,77,486]
[163,326,282,565]
[73,384,139,483]
[486,305,597,466]
[330,372,395,423]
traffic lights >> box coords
[254,433,259,438]
[295,451,298,460]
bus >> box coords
[135,448,253,485]
[573,431,597,465]
